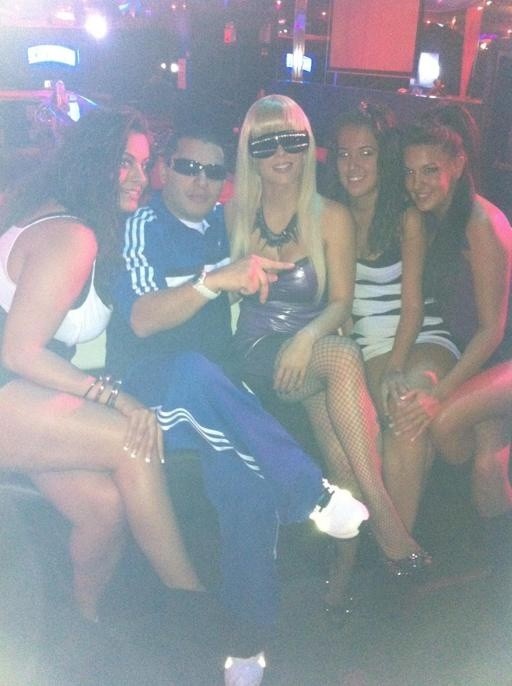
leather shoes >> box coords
[326,599,352,626]
[377,545,430,576]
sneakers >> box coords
[224,650,267,686]
[310,478,370,540]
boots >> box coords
[158,585,262,657]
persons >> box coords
[1,91,511,684]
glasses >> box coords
[248,131,310,158]
[172,157,224,181]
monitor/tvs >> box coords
[418,52,440,88]
[285,52,312,73]
